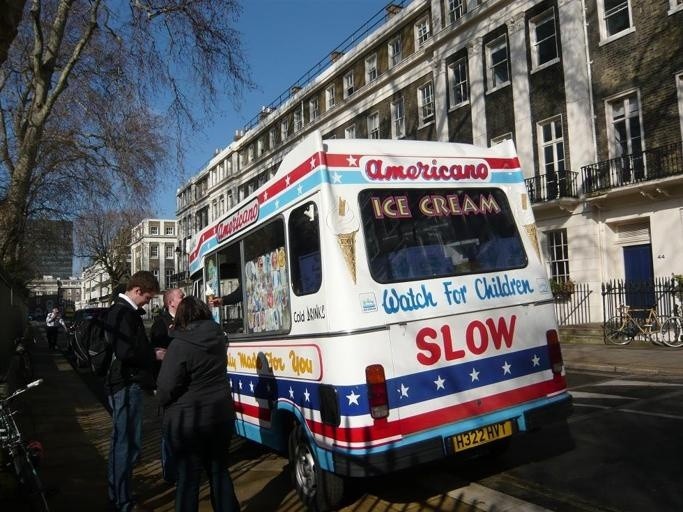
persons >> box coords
[144,293,243,512]
[148,286,185,484]
[208,244,262,307]
[102,269,169,512]
[44,306,68,353]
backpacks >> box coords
[60,307,117,383]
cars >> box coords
[67,308,109,368]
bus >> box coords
[188,129,573,512]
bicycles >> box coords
[0,378,63,512]
[8,336,33,382]
[604,304,683,348]
[29,326,41,344]
